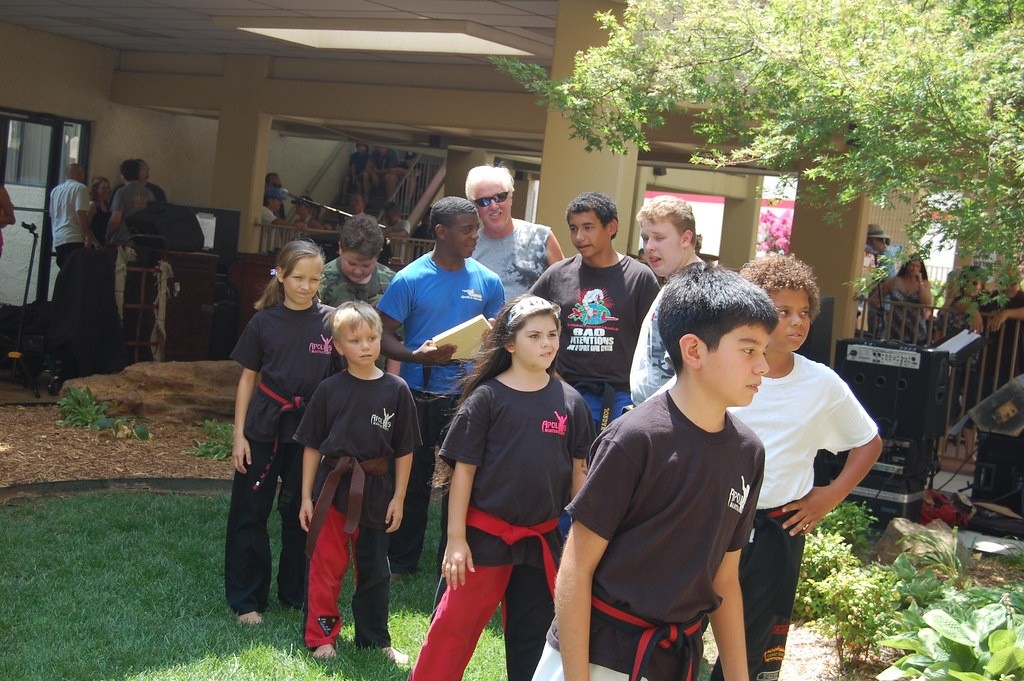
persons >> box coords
[225,235,349,623]
[317,212,404,377]
[339,142,423,217]
[530,261,781,681]
[408,292,598,681]
[260,173,342,254]
[866,221,1024,460]
[376,195,506,582]
[628,194,707,408]
[49,163,113,270]
[0,179,15,258]
[465,159,566,304]
[524,190,663,542]
[708,251,883,680]
[104,158,166,246]
[292,298,424,665]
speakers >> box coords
[971,432,1024,518]
[967,374,1024,436]
[835,338,949,443]
[125,203,204,252]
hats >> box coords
[264,187,287,200]
[866,223,890,239]
[292,195,314,208]
[405,151,417,160]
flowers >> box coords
[756,207,794,255]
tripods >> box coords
[0,222,41,397]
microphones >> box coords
[282,188,301,200]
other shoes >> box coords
[389,572,410,586]
[949,430,979,443]
[965,453,977,465]
[313,643,336,659]
[238,610,263,624]
[379,646,409,664]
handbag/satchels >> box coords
[125,202,205,252]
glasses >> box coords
[269,184,281,189]
[876,237,890,245]
[965,280,977,285]
[474,192,509,207]
